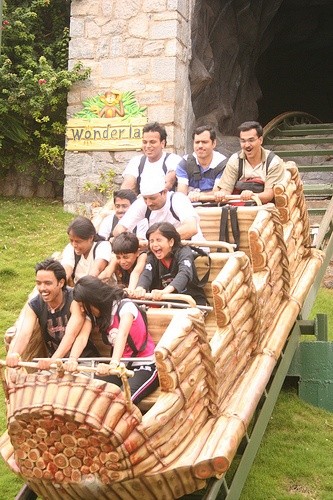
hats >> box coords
[140,168,166,196]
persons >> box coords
[129,221,211,318]
[5,258,86,372]
[214,121,287,207]
[175,124,228,203]
[96,232,148,298]
[55,216,118,287]
[98,189,149,242]
[112,168,210,253]
[118,122,180,192]
[61,275,160,406]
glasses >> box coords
[240,137,259,143]
